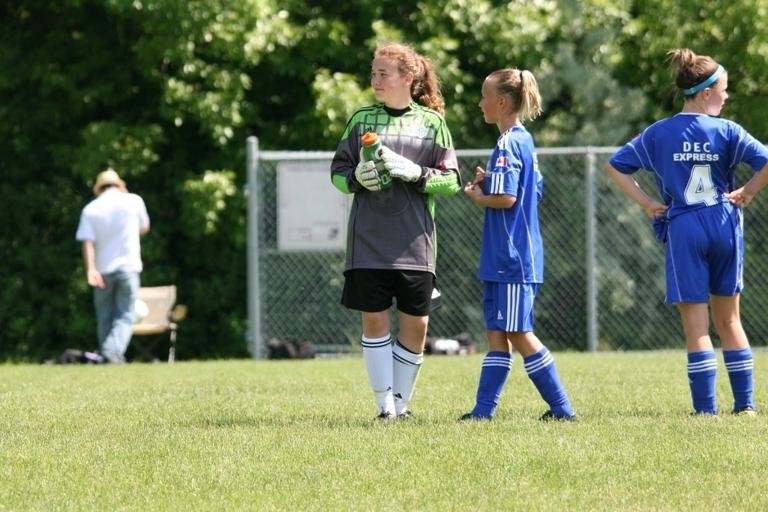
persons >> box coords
[75,166,151,362]
[328,43,464,430]
[458,69,580,426]
[604,46,768,413]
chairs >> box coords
[132,282,183,366]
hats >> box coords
[93,170,126,196]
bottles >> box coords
[360,130,392,190]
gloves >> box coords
[374,143,422,182]
[354,146,381,191]
